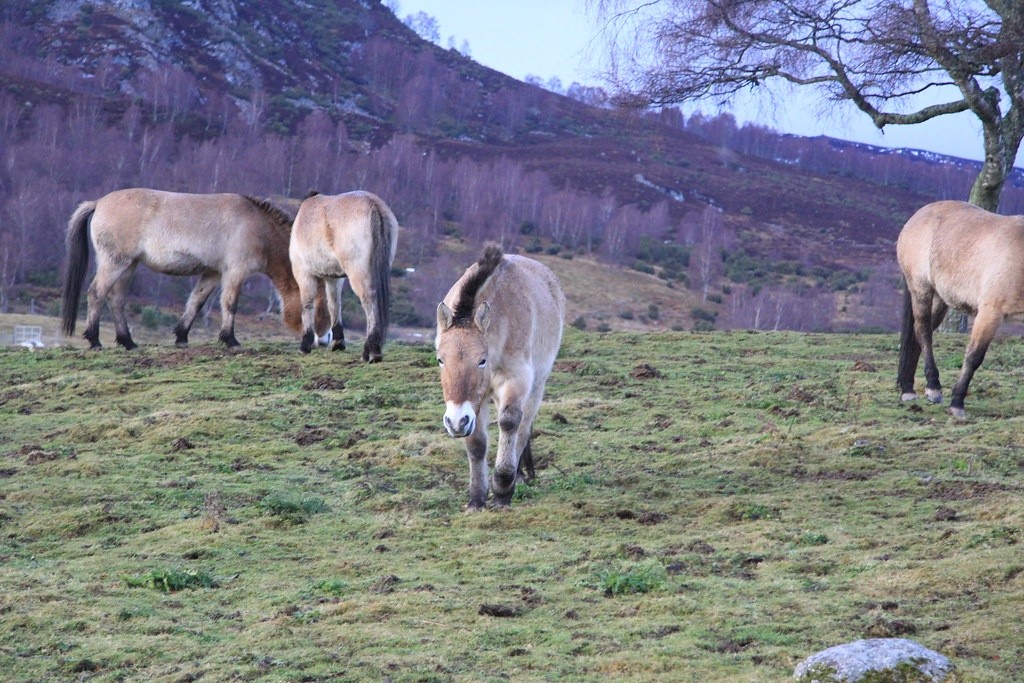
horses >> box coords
[289,190,399,364]
[435,245,566,518]
[894,198,1024,424]
[59,183,333,354]
